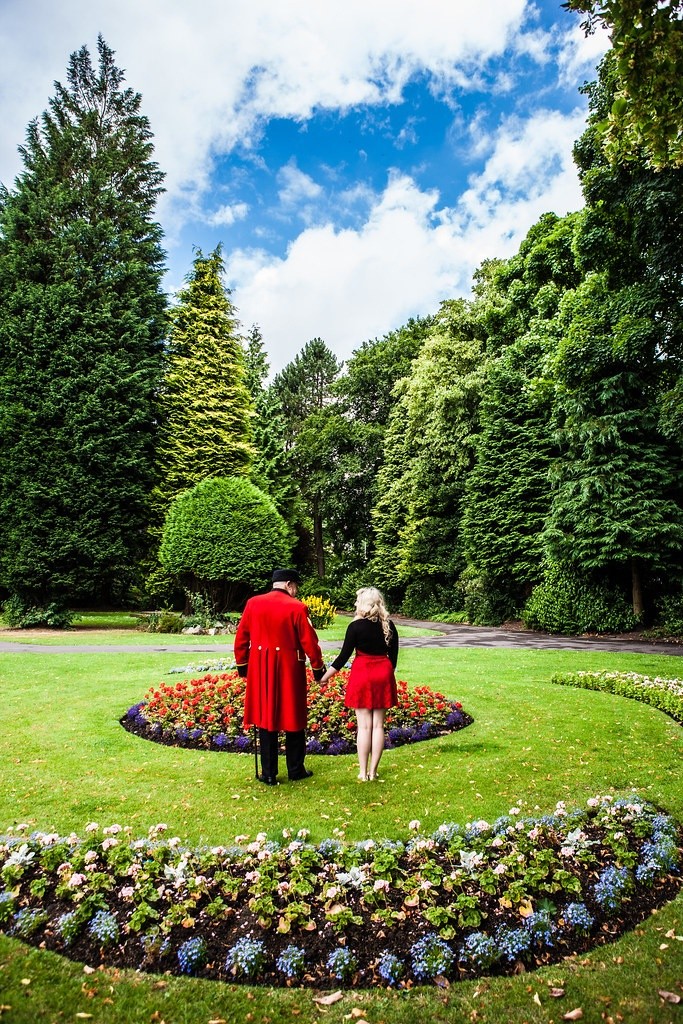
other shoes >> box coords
[358,773,379,782]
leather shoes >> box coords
[288,770,314,781]
[259,774,277,785]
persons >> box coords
[319,587,399,782]
[234,569,327,786]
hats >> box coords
[273,569,304,587]
